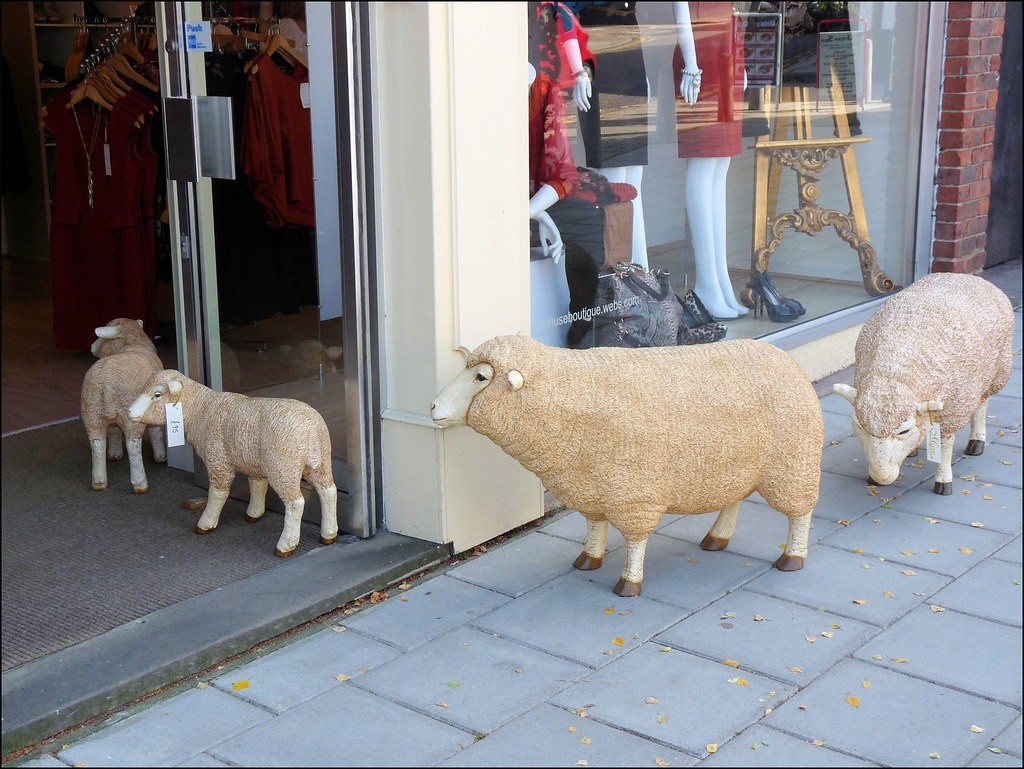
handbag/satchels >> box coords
[595,260,683,348]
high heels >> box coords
[683,290,727,339]
[751,272,799,323]
[674,293,715,345]
[762,272,806,316]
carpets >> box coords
[1,380,344,674]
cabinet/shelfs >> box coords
[0,0,138,263]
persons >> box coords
[671,0,750,320]
[528,0,653,352]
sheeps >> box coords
[430,333,824,598]
[79,318,165,492]
[124,369,339,556]
[831,271,1015,497]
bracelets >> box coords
[680,68,703,87]
[569,69,586,80]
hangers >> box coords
[214,16,308,81]
[37,16,159,130]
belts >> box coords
[587,4,636,17]
